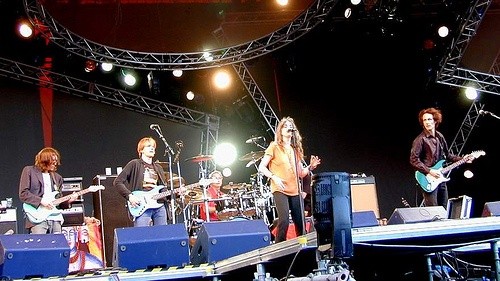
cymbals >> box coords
[163,172,185,187]
[239,151,265,161]
[185,154,213,162]
[154,161,174,168]
[245,137,262,144]
[223,184,239,190]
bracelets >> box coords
[270,174,274,179]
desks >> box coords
[60,220,105,273]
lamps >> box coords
[147,70,161,93]
[330,6,352,21]
[185,86,202,104]
[84,59,99,71]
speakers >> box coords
[351,211,379,227]
[482,202,500,216]
[350,184,380,218]
[190,219,271,265]
[113,223,190,270]
[387,206,447,224]
[0,234,70,278]
[92,176,135,269]
[310,172,353,258]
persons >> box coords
[299,175,312,217]
[19,147,78,234]
[410,108,473,210]
[200,171,225,221]
[113,137,187,227]
[259,117,320,243]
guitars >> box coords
[415,151,486,193]
[23,185,106,224]
[127,178,218,217]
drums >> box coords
[188,220,206,246]
[236,195,257,217]
[223,216,250,221]
[215,193,238,219]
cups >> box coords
[1,201,7,208]
[117,168,122,175]
[6,198,13,208]
[106,168,111,175]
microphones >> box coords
[245,136,262,143]
[150,124,158,130]
[288,128,297,131]
